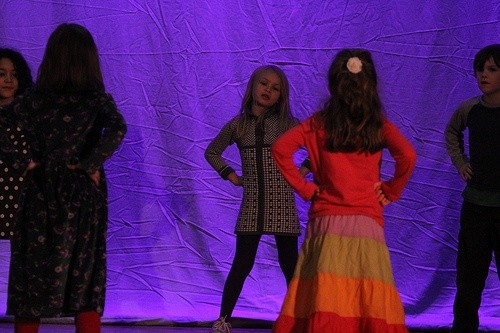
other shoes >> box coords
[210,316,232,333]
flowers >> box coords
[346,57,363,74]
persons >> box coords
[5,24,127,333]
[445,43,500,333]
[271,48,416,333]
[205,64,313,333]
[0,47,35,240]
